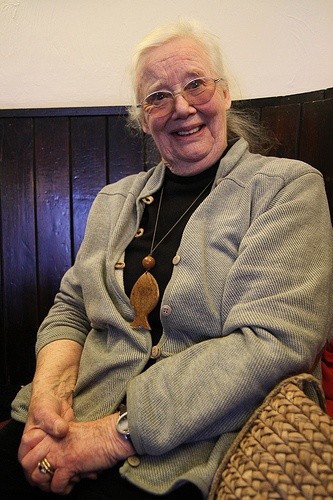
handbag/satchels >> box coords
[201,381,332,499]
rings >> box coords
[38,459,56,477]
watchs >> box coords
[116,404,137,452]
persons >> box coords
[0,17,333,500]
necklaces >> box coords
[130,170,217,330]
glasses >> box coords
[135,75,224,119]
